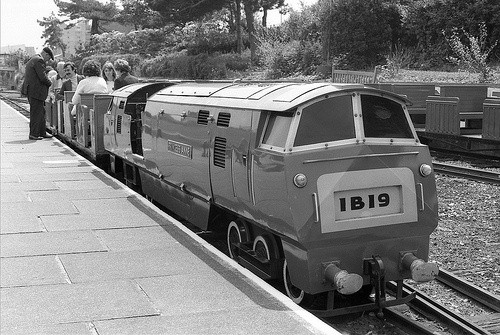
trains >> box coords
[45,71,439,319]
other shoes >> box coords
[30,135,43,139]
[40,132,53,137]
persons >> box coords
[44,66,57,78]
[20,47,54,140]
[114,59,139,91]
[56,61,86,101]
[101,60,116,93]
[71,60,109,136]
[48,61,69,102]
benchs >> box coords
[364,83,488,129]
[45,91,114,155]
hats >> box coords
[43,46,54,60]
